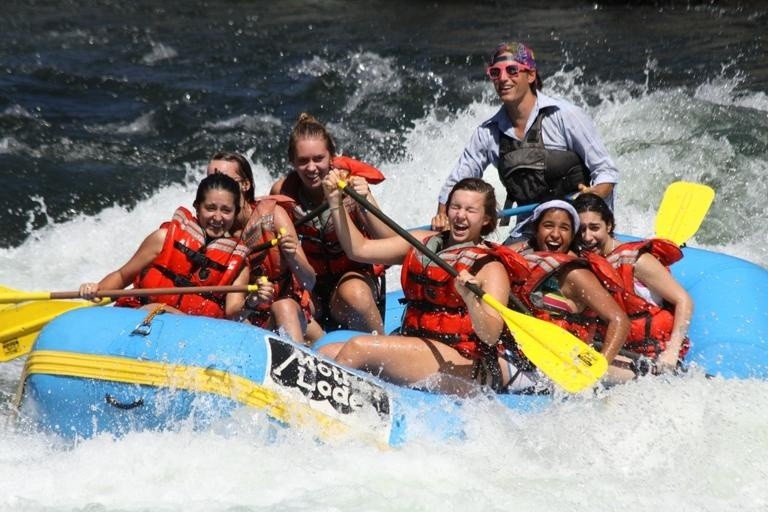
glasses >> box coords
[486,64,532,80]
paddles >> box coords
[337,181,608,394]
[655,181,714,251]
[0,237,278,361]
[0,278,269,309]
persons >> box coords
[77,172,275,320]
[268,113,385,346]
[496,198,631,396]
[207,151,326,348]
[429,42,620,256]
[571,193,693,389]
[314,168,530,402]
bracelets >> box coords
[329,204,343,211]
[244,298,257,310]
[360,205,369,214]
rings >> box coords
[272,288,274,293]
[87,287,91,289]
[440,222,444,224]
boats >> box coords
[13,232,768,450]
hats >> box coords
[488,40,544,92]
